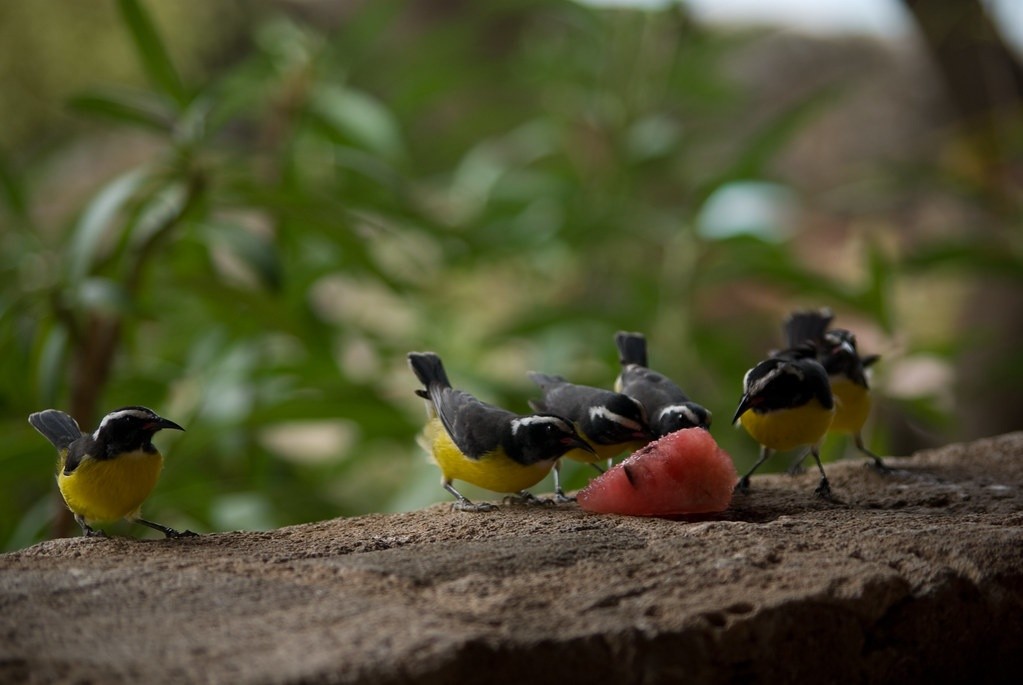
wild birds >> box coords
[784,311,894,481]
[612,331,714,452]
[523,367,658,503]
[405,349,600,514]
[27,404,201,542]
[729,354,839,502]
